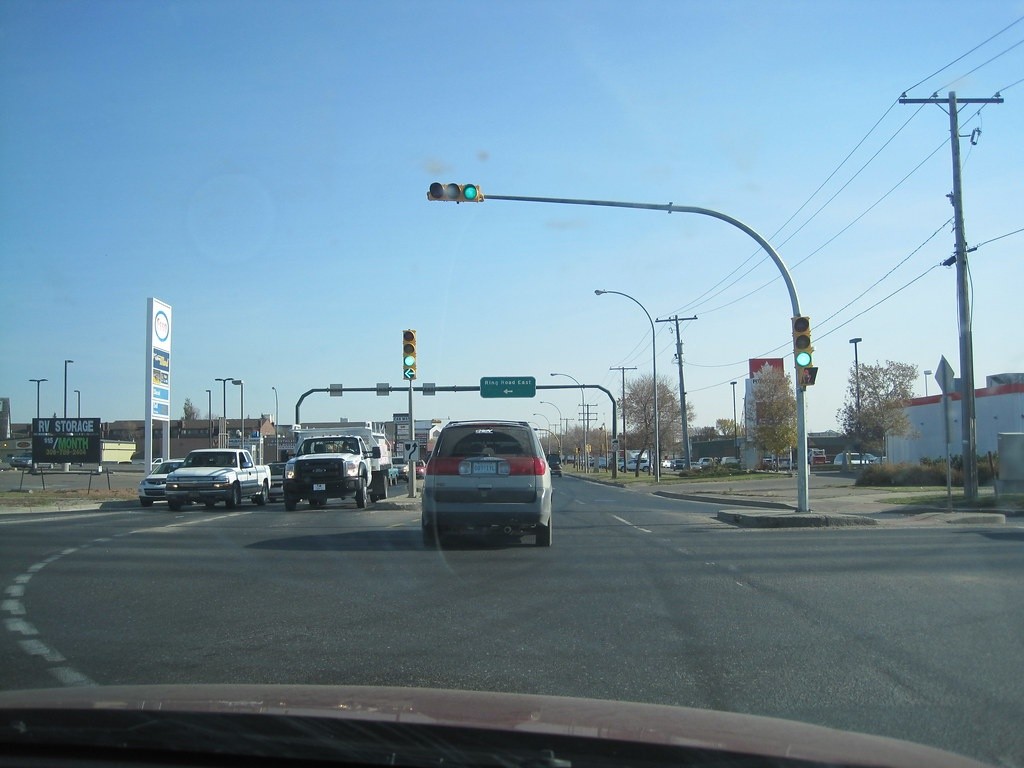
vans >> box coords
[833,452,886,466]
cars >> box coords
[138,457,192,506]
[545,453,562,477]
[265,463,287,502]
[9,450,54,470]
[392,456,427,482]
[574,456,714,473]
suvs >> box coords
[420,418,555,547]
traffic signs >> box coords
[479,376,536,398]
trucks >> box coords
[281,420,394,512]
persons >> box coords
[342,441,355,452]
[228,457,235,464]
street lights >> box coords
[215,378,234,432]
[231,380,245,450]
[849,337,862,468]
[271,387,279,462]
[29,378,48,419]
[540,401,562,466]
[550,372,588,474]
[74,390,81,419]
[205,389,213,448]
[924,370,933,397]
[64,359,73,418]
[595,289,660,483]
[533,413,551,455]
[730,381,738,459]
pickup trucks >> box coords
[163,448,272,511]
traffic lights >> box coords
[427,181,480,203]
[402,329,417,380]
[797,366,819,386]
[792,316,812,368]
